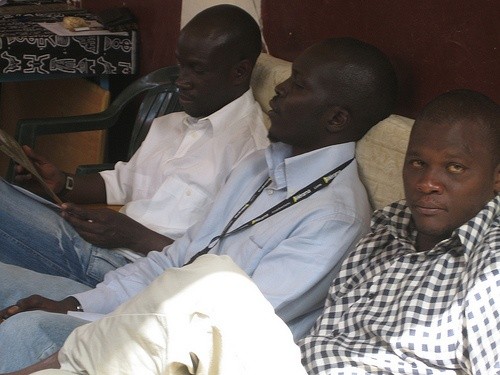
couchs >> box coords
[252,51,418,213]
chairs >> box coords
[3,63,187,193]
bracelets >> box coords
[67,296,83,313]
[56,171,74,199]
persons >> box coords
[1,88,500,375]
[1,35,399,375]
[0,4,270,290]
